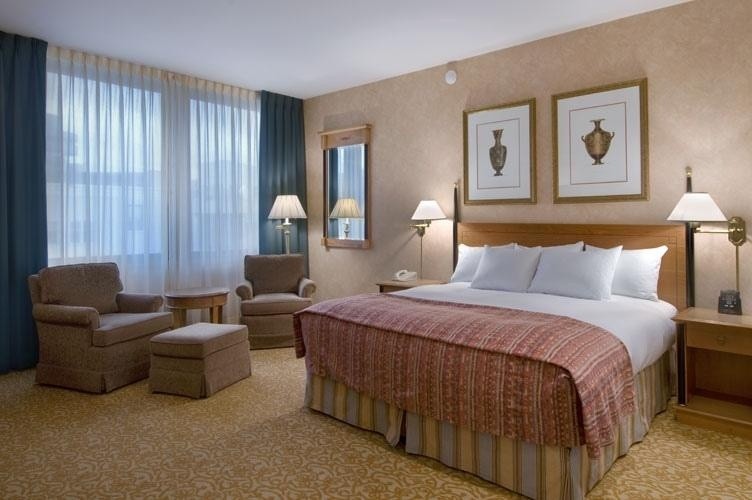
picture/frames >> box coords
[462,96,537,206]
[550,77,650,204]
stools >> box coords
[150,321,252,399]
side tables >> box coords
[163,291,229,328]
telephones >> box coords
[392,269,417,282]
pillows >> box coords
[514,239,584,251]
[528,244,623,303]
[584,245,669,302]
[467,245,542,293]
[450,242,517,283]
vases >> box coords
[489,129,507,176]
[581,118,614,165]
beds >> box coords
[292,166,696,500]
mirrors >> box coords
[316,123,371,249]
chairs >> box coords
[235,253,317,350]
[28,263,173,394]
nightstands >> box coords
[375,278,448,293]
[670,306,752,442]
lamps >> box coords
[664,193,746,246]
[329,198,362,238]
[267,194,308,253]
[409,200,447,237]
